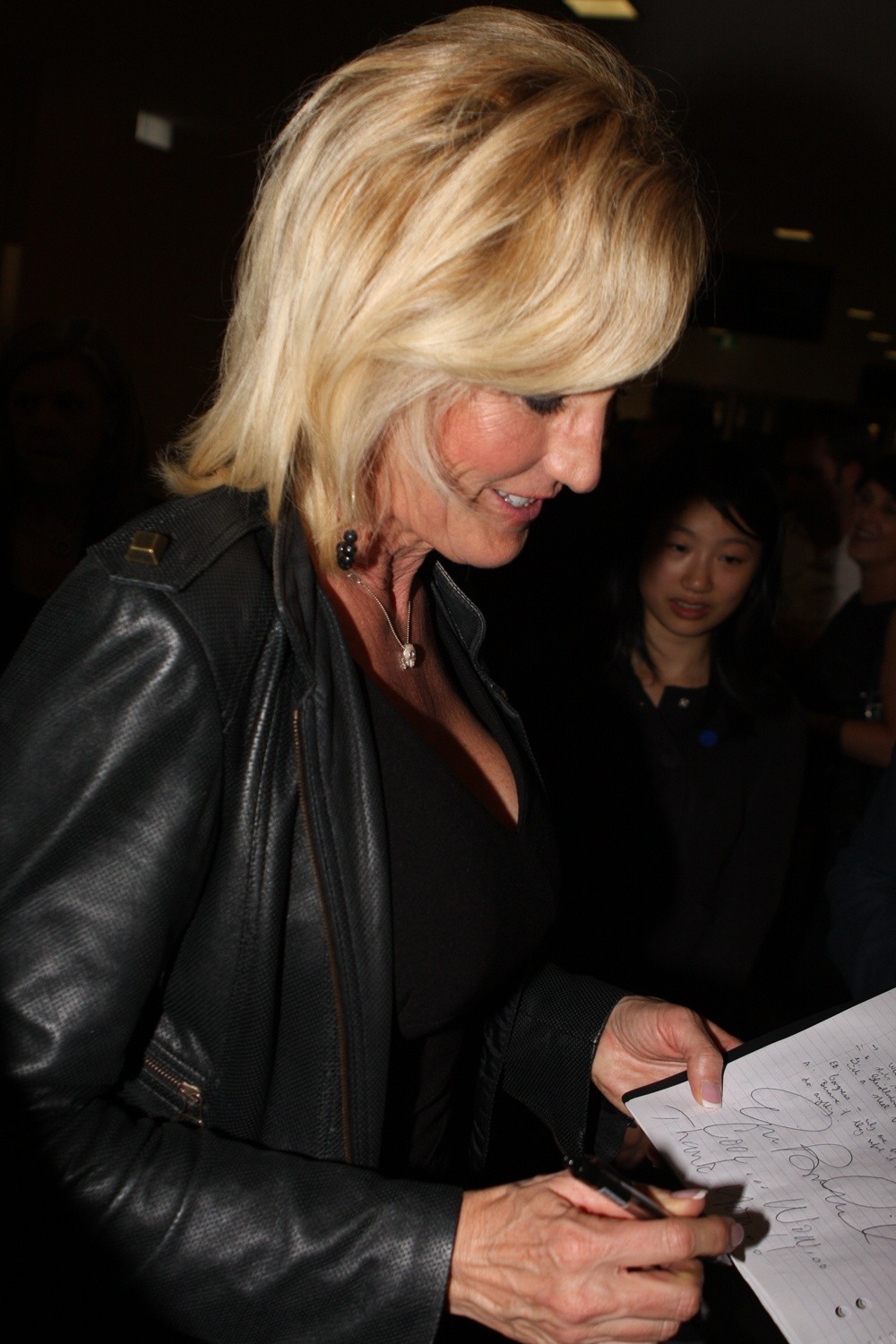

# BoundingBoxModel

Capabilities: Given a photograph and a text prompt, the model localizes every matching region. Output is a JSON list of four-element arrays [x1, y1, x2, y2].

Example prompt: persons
[[0, 319, 150, 663], [537, 412, 896, 1032], [0, 10, 745, 1344]]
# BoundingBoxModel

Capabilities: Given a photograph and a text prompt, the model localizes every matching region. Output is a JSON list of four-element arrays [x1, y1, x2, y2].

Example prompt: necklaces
[[348, 573, 416, 671]]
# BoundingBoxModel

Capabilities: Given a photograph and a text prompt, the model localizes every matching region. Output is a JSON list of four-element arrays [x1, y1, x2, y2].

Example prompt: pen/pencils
[[564, 1154, 732, 1266]]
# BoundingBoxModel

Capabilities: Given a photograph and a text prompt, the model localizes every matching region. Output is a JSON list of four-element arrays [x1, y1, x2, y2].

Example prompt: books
[[621, 983, 896, 1344]]
